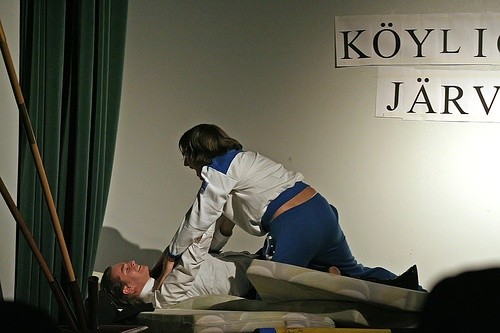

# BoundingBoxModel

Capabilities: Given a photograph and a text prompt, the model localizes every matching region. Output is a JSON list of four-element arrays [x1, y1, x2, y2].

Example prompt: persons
[[152, 123, 430, 292], [101, 204, 339, 302]]
